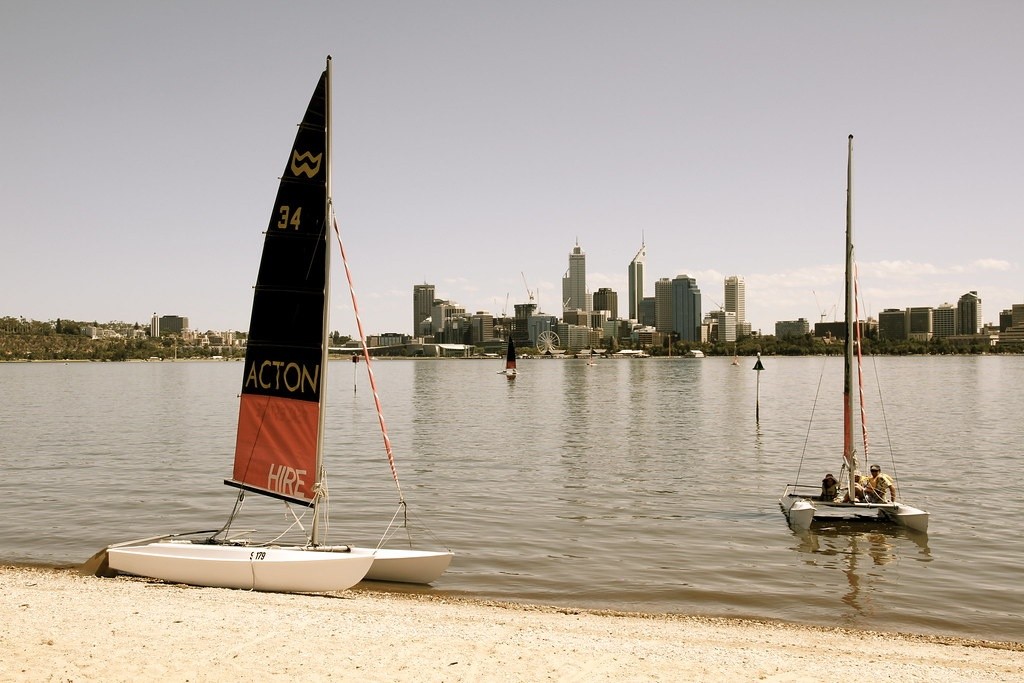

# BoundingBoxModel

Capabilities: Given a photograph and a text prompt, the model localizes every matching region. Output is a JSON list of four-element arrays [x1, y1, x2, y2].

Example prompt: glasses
[[871, 470, 878, 472]]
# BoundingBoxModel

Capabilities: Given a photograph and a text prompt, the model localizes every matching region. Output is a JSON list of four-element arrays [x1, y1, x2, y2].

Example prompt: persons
[[820, 464, 896, 504]]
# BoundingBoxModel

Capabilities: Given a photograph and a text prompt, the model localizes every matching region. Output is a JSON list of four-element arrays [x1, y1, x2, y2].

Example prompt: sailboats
[[496, 334, 518, 380], [85, 52, 458, 591], [777, 133, 934, 542]]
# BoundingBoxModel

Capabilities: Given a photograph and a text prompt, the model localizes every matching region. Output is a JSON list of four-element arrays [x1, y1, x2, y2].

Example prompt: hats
[[870, 465, 881, 471], [822, 474, 837, 485]]
